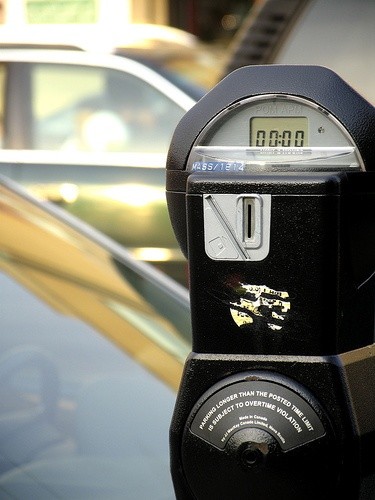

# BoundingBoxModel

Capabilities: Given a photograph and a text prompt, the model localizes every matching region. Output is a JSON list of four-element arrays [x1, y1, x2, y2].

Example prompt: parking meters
[[166, 65, 374, 500]]
